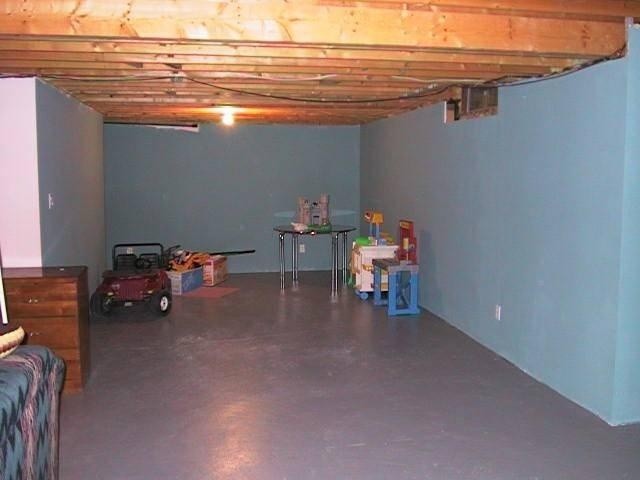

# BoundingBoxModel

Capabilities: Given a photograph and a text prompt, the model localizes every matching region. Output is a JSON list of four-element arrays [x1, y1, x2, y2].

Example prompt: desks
[[273, 226, 356, 298]]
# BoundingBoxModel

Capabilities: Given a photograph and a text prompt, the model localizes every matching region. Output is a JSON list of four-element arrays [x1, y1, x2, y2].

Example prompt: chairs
[[116, 254, 160, 271]]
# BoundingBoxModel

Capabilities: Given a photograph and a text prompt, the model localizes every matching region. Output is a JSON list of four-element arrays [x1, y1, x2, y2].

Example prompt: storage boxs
[[166, 254, 227, 295]]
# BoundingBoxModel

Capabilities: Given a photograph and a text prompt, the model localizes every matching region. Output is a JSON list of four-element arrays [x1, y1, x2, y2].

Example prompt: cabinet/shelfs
[[1, 267, 90, 395]]
